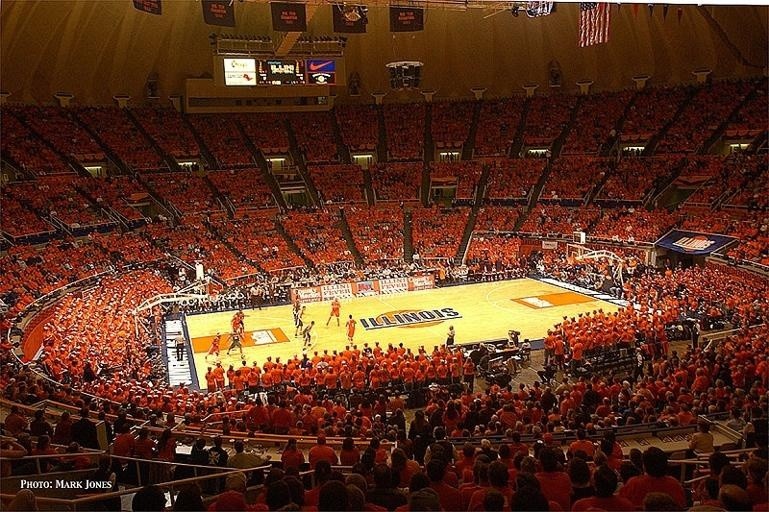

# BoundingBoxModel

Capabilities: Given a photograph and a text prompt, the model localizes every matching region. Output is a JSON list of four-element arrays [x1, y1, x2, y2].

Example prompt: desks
[[455, 338, 519, 357]]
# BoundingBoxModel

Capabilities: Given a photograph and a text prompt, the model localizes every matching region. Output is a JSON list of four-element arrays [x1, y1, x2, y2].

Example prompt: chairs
[[584, 346, 669, 375], [520, 347, 531, 362], [477, 356, 504, 381]]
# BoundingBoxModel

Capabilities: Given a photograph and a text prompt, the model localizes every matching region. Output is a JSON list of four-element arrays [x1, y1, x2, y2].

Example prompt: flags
[[577, 2, 611, 48]]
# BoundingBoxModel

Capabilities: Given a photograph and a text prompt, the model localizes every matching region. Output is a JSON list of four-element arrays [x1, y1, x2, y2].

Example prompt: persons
[[1, 74, 769, 512]]
